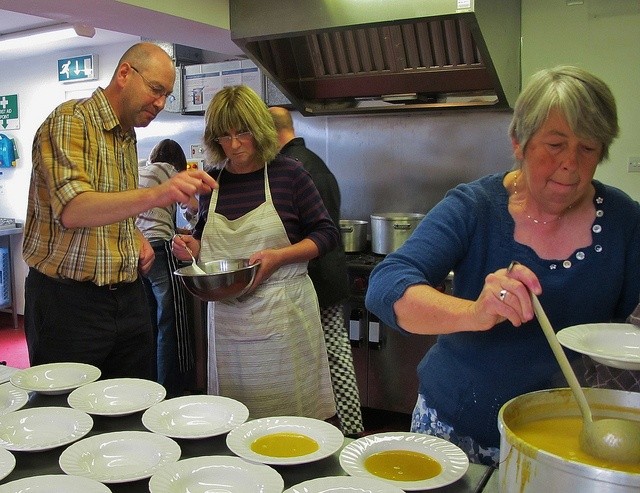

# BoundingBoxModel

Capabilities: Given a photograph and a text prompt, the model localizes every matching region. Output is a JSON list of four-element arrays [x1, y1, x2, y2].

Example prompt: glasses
[[129, 65, 176, 103], [216, 130, 252, 144]]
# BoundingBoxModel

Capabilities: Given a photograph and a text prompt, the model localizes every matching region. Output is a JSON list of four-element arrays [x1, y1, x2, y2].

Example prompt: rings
[[498, 288, 509, 301]]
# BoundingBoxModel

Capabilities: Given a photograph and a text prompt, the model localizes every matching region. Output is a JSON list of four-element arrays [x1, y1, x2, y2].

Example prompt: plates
[[142, 394, 250, 439], [0, 406, 94, 453], [149, 455, 285, 492], [0, 447, 17, 481], [59, 431, 182, 482], [283, 476, 406, 493], [11, 361, 101, 396], [225, 416, 345, 466], [339, 431, 469, 491], [0, 382, 29, 412], [1, 474, 111, 493], [556, 322, 640, 370], [68, 378, 167, 416]]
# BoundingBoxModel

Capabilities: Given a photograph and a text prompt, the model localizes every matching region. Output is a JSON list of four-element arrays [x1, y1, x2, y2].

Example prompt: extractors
[[228, 0, 523, 118]]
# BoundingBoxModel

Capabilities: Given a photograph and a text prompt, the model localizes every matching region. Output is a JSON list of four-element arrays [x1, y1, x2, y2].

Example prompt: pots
[[338, 218, 370, 254], [370, 213, 424, 256], [498, 387, 640, 493]]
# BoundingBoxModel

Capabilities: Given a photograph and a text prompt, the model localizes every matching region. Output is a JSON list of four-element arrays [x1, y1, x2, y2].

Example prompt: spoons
[[526, 287, 640, 462], [176, 234, 206, 274]]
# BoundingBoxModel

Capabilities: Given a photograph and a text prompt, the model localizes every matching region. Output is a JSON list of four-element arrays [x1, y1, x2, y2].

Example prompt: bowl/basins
[[174, 258, 262, 302]]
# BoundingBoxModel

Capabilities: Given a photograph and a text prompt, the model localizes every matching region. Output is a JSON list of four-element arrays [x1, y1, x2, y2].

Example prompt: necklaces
[[520, 202, 576, 225]]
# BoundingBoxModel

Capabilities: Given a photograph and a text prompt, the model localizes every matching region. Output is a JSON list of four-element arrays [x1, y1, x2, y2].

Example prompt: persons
[[265, 105, 364, 438], [137, 137, 200, 393], [23, 41, 177, 382], [171, 85, 343, 427], [363, 66, 640, 468]]
